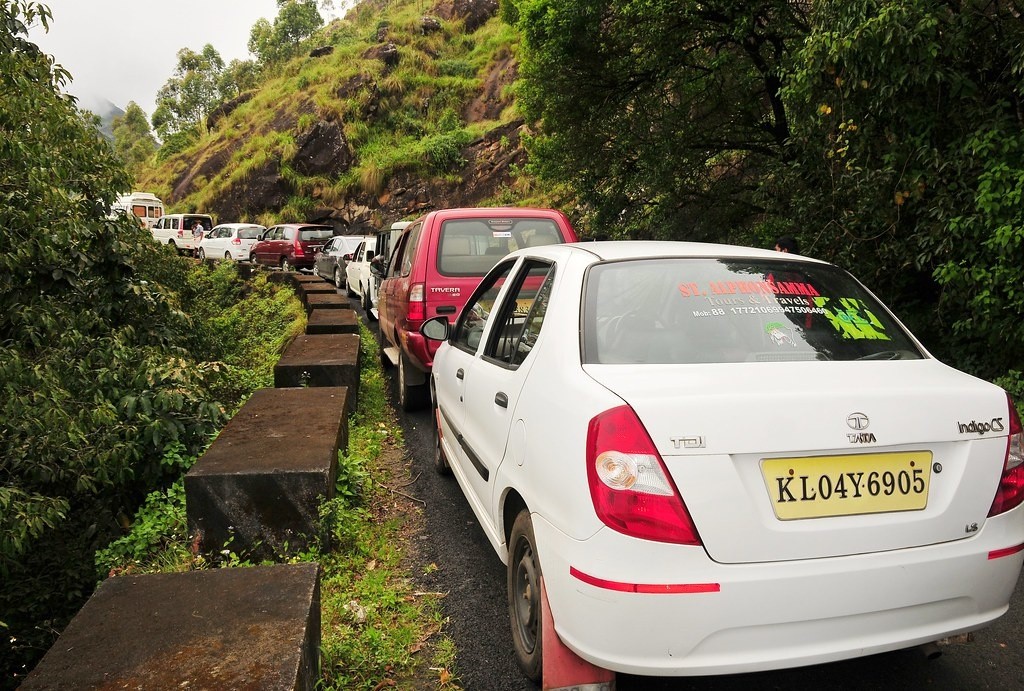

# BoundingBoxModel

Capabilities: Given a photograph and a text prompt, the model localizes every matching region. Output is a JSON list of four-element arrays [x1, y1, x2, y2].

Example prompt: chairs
[[486, 247, 510, 255]]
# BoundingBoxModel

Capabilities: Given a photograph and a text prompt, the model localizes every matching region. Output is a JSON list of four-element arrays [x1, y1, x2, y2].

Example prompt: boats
[[419, 242, 1024, 691]]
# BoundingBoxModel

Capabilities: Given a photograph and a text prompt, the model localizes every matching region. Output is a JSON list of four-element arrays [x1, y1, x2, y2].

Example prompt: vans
[[368, 208, 579, 416]]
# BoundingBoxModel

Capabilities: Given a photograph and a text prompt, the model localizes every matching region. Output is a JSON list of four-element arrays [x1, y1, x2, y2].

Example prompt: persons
[[372, 254, 380, 262], [191, 220, 203, 258]]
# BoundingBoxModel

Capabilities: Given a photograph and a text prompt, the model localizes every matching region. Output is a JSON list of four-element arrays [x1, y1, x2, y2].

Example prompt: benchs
[[442, 235, 560, 272]]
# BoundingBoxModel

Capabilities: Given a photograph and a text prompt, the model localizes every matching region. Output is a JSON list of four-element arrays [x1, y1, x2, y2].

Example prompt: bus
[[96, 191, 165, 229]]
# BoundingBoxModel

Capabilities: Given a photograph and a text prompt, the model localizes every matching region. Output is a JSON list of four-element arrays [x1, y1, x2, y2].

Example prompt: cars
[[149, 213, 215, 254], [198, 223, 267, 262], [342, 237, 379, 310], [312, 235, 376, 288], [368, 221, 415, 317], [250, 223, 335, 269]]
